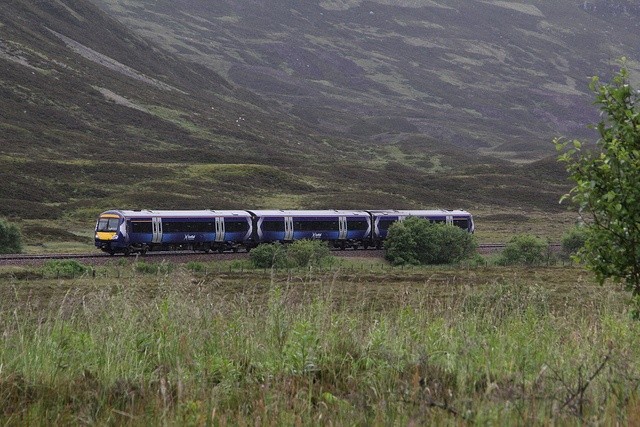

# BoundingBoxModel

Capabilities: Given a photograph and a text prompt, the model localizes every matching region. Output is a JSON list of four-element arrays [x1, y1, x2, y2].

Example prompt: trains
[[92, 207, 479, 258]]
